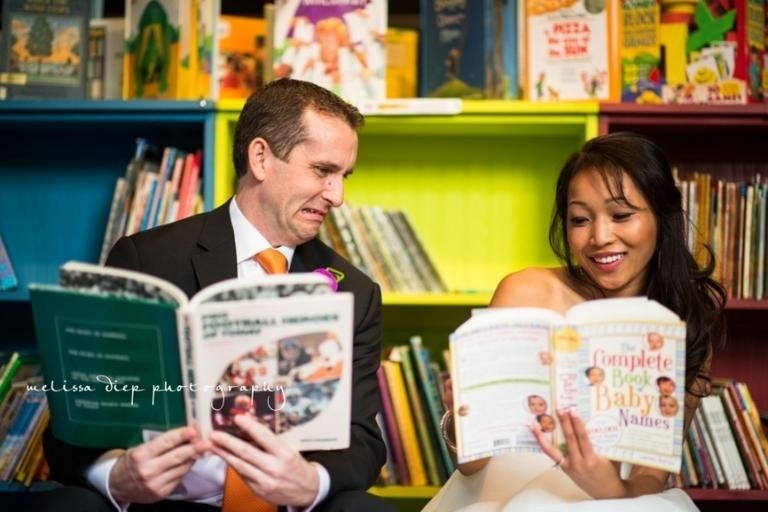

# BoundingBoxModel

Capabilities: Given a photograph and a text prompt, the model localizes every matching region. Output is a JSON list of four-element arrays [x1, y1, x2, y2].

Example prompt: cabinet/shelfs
[[0, 95, 767, 512]]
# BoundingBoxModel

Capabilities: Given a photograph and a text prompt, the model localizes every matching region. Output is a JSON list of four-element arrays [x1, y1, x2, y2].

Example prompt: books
[[326, 205, 448, 295], [23, 261, 352, 453], [1, 350, 48, 484], [671, 381, 768, 491], [667, 160, 768, 299], [100, 138, 203, 267], [448, 296, 687, 478], [0, 1, 767, 105], [378, 332, 455, 487]]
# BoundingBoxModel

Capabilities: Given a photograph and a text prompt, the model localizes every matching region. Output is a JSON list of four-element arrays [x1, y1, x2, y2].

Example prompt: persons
[[527, 333, 678, 431], [41, 79, 387, 512], [425, 130, 721, 512]]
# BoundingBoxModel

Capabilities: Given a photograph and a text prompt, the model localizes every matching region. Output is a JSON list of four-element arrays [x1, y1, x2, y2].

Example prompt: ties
[[222, 251, 289, 510]]
